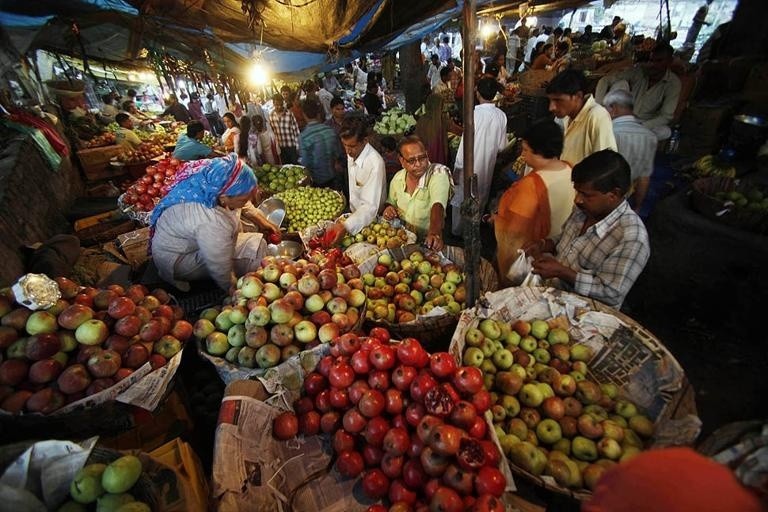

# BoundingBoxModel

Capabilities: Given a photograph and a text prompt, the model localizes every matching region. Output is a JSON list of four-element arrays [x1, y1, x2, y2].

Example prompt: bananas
[[692, 154, 737, 179]]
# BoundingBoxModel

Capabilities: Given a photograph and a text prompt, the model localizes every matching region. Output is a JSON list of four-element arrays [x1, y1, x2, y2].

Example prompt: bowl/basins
[[45, 78, 86, 98], [255, 197, 289, 234], [266, 241, 303, 265], [159, 120, 172, 127]]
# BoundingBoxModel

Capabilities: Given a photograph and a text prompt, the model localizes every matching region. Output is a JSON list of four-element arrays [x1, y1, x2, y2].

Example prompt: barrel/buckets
[[446, 285, 702, 501], [360, 239, 499, 345], [257, 198, 289, 229], [277, 240, 304, 260]]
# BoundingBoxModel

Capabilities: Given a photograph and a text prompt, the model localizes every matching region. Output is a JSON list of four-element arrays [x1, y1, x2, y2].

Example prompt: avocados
[[55, 454, 151, 512]]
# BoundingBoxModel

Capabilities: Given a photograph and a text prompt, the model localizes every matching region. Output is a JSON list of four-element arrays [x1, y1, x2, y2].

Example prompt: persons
[[147, 153, 284, 296], [158, 16, 698, 313], [123, 101, 154, 128], [108, 92, 122, 108], [142, 92, 150, 103], [114, 113, 152, 142], [101, 95, 120, 117], [120, 89, 137, 107]]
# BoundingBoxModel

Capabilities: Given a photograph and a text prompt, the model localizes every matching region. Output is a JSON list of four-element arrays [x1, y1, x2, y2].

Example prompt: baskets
[[365, 313, 456, 350]]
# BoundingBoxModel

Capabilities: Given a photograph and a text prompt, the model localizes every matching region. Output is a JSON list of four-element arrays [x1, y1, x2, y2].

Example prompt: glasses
[[400, 153, 427, 164]]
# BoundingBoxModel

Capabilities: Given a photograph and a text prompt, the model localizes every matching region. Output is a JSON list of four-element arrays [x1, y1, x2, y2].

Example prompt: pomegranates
[[121, 158, 189, 210]]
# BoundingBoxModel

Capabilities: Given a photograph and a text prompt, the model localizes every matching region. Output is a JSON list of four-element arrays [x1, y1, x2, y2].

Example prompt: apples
[[271, 186, 467, 323], [463, 318, 651, 489], [194, 248, 367, 368], [274, 327, 506, 511], [1, 276, 193, 412]]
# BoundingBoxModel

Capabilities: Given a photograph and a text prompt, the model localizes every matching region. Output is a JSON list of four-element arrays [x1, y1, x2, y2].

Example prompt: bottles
[[664, 123, 681, 155]]
